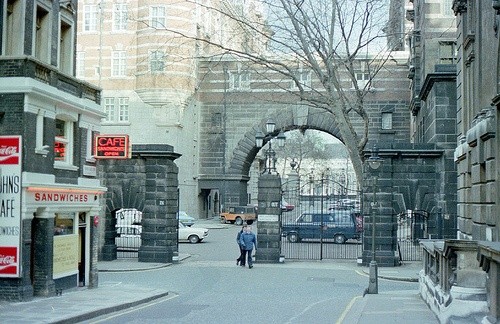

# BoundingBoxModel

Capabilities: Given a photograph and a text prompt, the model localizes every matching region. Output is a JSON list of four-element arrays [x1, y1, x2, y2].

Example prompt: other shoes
[[236, 259, 239, 265], [242, 265, 245, 267], [249, 265, 253, 269]]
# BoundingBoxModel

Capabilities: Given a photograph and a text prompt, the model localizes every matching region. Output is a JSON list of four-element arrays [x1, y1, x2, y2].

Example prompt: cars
[[329, 199, 361, 211], [281, 200, 294, 212], [179, 211, 209, 244], [115, 224, 142, 252]]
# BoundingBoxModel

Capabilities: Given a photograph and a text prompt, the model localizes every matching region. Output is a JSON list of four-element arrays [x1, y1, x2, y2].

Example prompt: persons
[[236, 227, 246, 266], [240, 226, 257, 268]]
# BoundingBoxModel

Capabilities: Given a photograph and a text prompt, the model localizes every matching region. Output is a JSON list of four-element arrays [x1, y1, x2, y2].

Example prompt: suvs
[[220, 206, 256, 226], [282, 210, 361, 244]]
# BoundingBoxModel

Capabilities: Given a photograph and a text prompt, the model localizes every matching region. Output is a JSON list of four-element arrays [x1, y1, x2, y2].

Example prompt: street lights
[[365, 145, 384, 294]]
